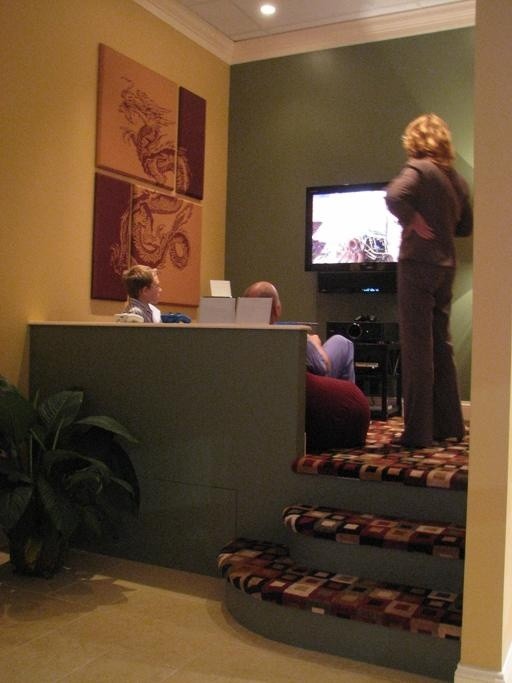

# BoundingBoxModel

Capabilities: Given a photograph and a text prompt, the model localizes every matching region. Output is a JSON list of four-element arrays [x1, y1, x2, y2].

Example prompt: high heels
[[390, 424, 466, 449]]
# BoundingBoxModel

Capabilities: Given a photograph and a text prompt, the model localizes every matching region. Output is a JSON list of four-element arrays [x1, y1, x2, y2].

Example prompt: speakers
[[324, 320, 400, 343]]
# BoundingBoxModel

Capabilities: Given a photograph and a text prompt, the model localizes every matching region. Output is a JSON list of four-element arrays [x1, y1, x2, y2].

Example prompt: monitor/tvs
[[304, 182, 404, 275]]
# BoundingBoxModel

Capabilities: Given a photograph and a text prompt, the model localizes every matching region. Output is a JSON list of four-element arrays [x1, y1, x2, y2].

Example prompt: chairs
[[306, 371, 371, 450]]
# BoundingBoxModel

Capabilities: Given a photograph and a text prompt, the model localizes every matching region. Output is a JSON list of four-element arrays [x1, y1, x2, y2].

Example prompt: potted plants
[[0, 377, 145, 578]]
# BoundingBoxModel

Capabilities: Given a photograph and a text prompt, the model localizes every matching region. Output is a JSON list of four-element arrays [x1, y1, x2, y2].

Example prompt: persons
[[122, 263, 165, 323], [381, 111, 475, 450], [240, 279, 356, 386]]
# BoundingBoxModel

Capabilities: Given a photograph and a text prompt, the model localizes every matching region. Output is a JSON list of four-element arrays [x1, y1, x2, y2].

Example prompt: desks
[[355, 342, 402, 421]]
